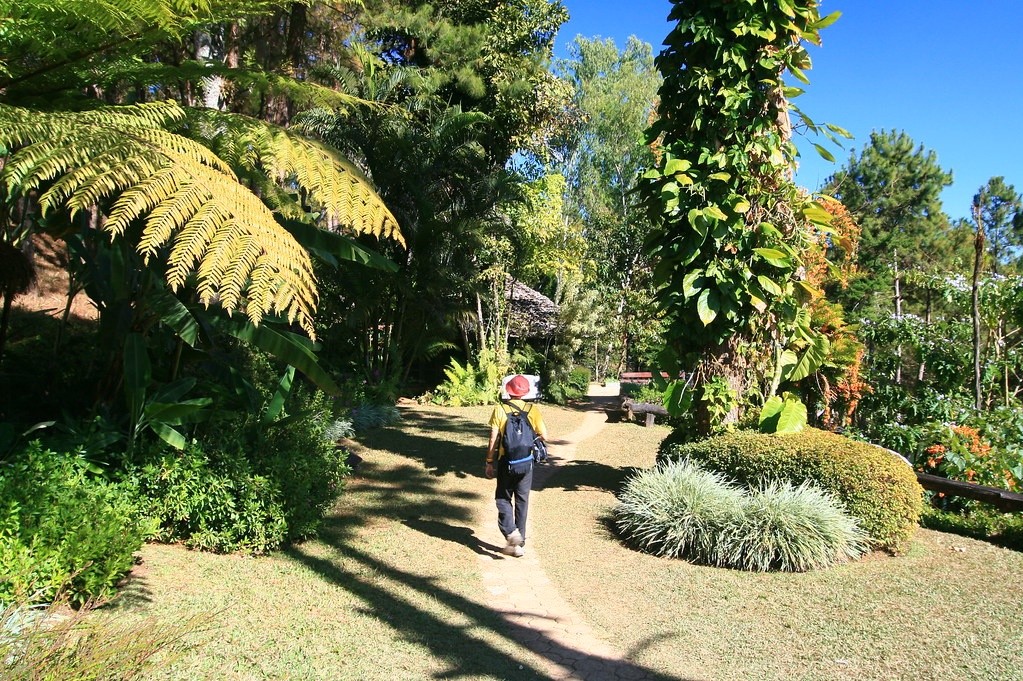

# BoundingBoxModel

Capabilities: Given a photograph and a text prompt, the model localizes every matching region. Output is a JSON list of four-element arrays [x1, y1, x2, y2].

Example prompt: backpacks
[[501, 402, 533, 477]]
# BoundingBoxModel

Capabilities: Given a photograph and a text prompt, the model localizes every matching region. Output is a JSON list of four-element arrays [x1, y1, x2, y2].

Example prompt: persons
[[485, 375, 547, 556]]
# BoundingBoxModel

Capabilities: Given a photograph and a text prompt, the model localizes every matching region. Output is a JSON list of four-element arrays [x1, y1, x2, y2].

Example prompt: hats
[[507, 374, 530, 396]]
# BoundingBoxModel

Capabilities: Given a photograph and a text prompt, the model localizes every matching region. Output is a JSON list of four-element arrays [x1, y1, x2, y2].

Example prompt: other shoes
[[514, 544, 525, 557], [502, 527, 522, 555]]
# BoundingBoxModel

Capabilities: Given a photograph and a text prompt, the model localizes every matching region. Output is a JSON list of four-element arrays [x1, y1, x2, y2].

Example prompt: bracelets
[[486, 461, 493, 464]]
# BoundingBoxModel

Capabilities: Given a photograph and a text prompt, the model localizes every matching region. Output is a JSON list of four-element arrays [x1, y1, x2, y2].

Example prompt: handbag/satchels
[[533, 433, 547, 464]]
